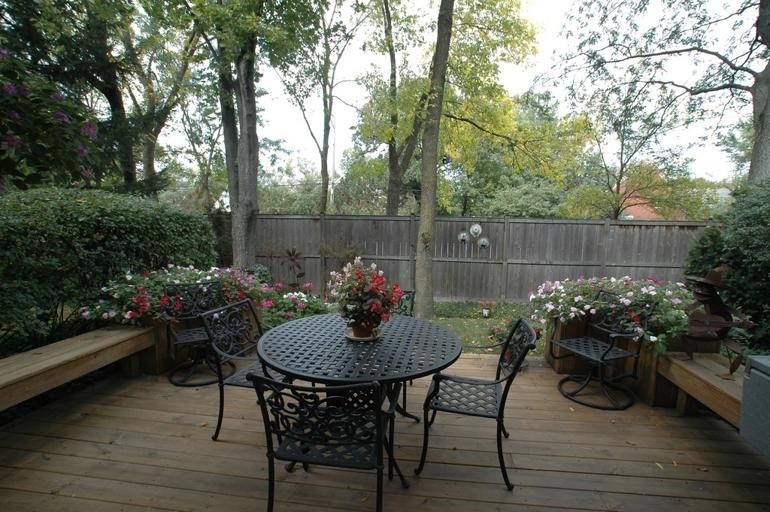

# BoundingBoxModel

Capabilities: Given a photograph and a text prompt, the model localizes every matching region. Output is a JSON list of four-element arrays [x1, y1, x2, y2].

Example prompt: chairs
[[244, 372, 409, 512], [549, 290, 658, 410], [161, 281, 239, 387], [389, 290, 416, 413], [413, 318, 536, 491], [198, 298, 296, 448]]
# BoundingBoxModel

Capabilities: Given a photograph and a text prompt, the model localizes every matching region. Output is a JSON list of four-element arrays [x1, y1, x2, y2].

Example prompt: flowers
[[326, 255, 405, 322]]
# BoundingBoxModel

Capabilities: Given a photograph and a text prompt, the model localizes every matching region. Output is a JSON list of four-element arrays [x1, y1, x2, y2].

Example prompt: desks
[[255, 313, 463, 489]]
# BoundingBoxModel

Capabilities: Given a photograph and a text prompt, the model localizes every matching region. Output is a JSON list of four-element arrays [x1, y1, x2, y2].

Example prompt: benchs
[[0, 324, 166, 415], [656, 352, 747, 431]]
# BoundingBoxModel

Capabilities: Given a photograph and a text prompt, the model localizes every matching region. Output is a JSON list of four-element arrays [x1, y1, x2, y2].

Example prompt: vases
[[343, 320, 384, 342]]
